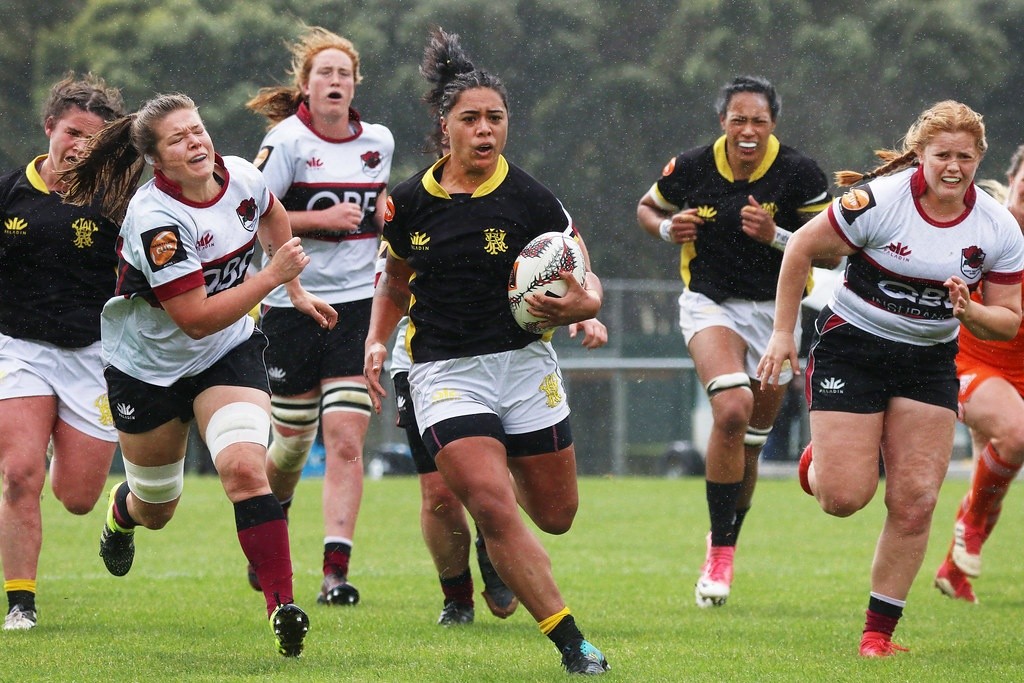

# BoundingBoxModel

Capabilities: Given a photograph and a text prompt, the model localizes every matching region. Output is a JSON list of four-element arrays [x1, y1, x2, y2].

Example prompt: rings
[[546, 314, 550, 319]]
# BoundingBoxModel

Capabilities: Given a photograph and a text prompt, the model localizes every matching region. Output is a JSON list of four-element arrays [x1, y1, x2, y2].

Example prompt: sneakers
[[438, 602, 475, 626], [953, 521, 989, 577], [1, 603, 38, 632], [318, 573, 358, 607], [45, 437, 54, 461], [473, 523, 520, 619], [934, 561, 980, 604], [270, 592, 309, 656], [798, 443, 813, 496], [857, 633, 909, 659], [560, 639, 611, 676], [100, 481, 136, 576], [695, 533, 737, 607], [247, 563, 263, 592]]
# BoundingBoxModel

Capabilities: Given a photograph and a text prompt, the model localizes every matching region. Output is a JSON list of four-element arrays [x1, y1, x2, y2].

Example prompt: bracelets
[[660, 220, 672, 242], [771, 226, 793, 252]]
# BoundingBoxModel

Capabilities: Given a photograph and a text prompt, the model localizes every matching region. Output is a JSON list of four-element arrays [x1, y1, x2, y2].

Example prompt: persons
[[935, 148, 1024, 602], [391, 314, 607, 624], [361, 29, 609, 676], [637, 76, 840, 605], [755, 101, 1024, 657], [0, 73, 126, 629], [54, 94, 337, 656], [249, 30, 395, 606]]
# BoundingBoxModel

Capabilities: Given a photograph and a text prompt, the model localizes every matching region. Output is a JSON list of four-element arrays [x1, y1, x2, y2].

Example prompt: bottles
[[368, 453, 384, 480]]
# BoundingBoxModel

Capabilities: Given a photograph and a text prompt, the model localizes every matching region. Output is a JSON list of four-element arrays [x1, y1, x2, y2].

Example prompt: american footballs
[[508, 227, 584, 334]]
